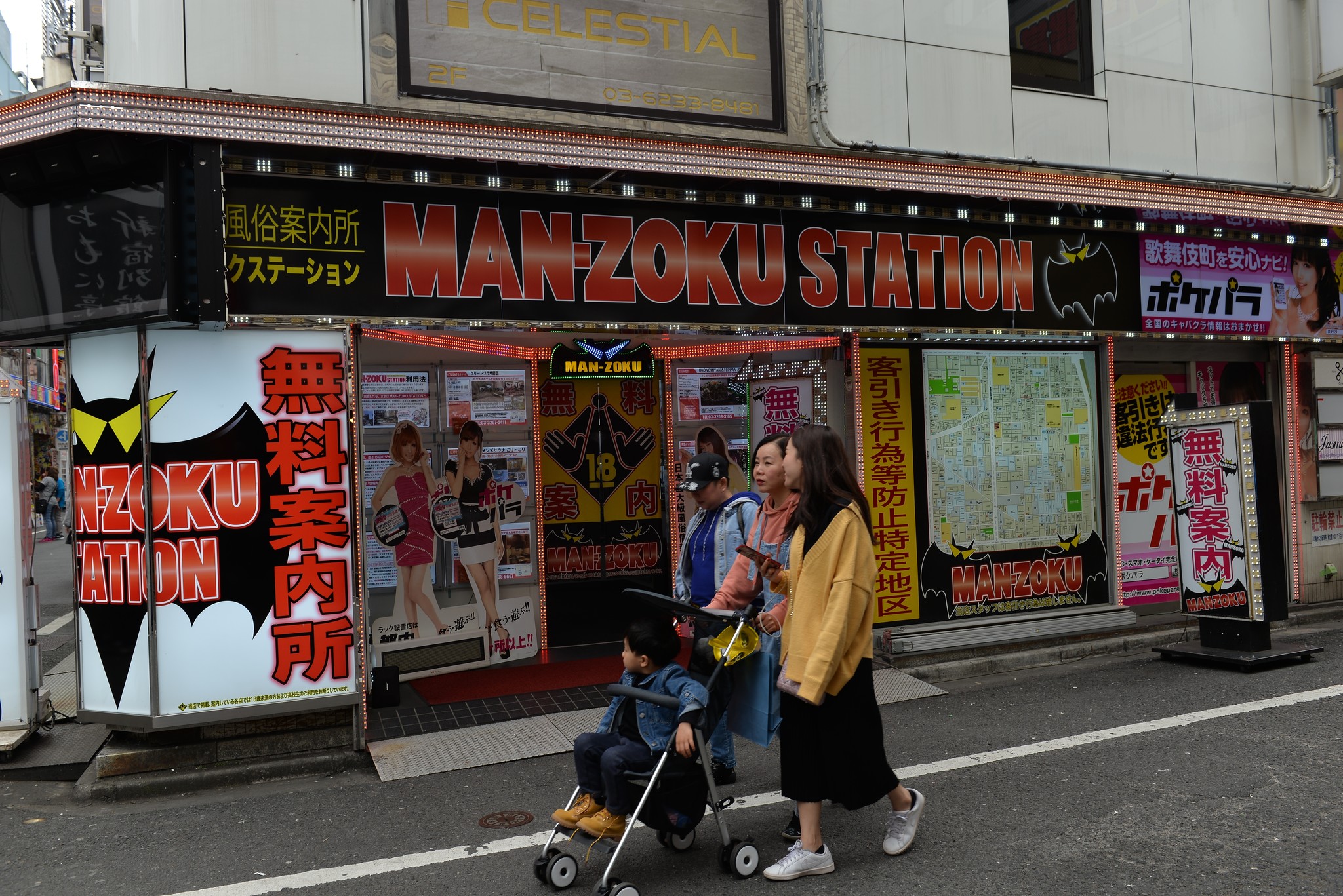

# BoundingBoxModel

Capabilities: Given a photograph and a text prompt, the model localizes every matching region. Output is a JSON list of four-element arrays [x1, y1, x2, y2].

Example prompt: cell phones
[[735, 544, 781, 570]]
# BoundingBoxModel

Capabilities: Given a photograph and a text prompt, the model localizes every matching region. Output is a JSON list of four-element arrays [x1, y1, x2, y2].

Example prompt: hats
[[674, 452, 729, 492]]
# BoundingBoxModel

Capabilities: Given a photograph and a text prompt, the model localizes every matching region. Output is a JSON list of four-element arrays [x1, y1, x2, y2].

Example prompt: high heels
[[498, 628, 510, 660], [488, 634, 492, 657]]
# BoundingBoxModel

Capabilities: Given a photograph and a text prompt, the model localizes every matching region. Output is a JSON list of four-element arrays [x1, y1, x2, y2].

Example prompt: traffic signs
[[55, 427, 68, 451]]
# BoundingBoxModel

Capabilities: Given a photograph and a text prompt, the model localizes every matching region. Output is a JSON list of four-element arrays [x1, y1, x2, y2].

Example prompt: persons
[[552, 618, 708, 840], [51, 467, 65, 540], [371, 417, 451, 640], [1264, 246, 1343, 336], [675, 452, 765, 785], [1296, 364, 1318, 501], [30, 465, 58, 543], [699, 433, 802, 840], [63, 481, 72, 533], [756, 422, 925, 879], [444, 419, 512, 662]]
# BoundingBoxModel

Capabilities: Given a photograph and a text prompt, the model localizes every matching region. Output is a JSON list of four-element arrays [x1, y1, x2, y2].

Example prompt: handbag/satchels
[[65, 529, 72, 545], [777, 651, 826, 707], [35, 498, 48, 514]]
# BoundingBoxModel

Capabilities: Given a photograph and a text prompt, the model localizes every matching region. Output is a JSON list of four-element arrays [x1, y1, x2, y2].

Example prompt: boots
[[551, 792, 605, 830], [576, 807, 626, 842]]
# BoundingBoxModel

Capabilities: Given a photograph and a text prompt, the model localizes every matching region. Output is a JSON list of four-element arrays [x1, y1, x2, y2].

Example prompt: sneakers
[[39, 536, 56, 543], [781, 810, 802, 839], [882, 787, 925, 856], [55, 532, 64, 540], [709, 758, 736, 785], [764, 839, 835, 880]]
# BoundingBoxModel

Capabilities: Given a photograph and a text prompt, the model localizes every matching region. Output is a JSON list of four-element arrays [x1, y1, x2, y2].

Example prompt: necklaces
[[401, 463, 416, 473], [1297, 298, 1318, 323], [1299, 449, 1308, 477]]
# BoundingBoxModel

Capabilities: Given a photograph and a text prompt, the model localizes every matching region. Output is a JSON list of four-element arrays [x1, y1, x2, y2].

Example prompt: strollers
[[533, 587, 765, 896]]
